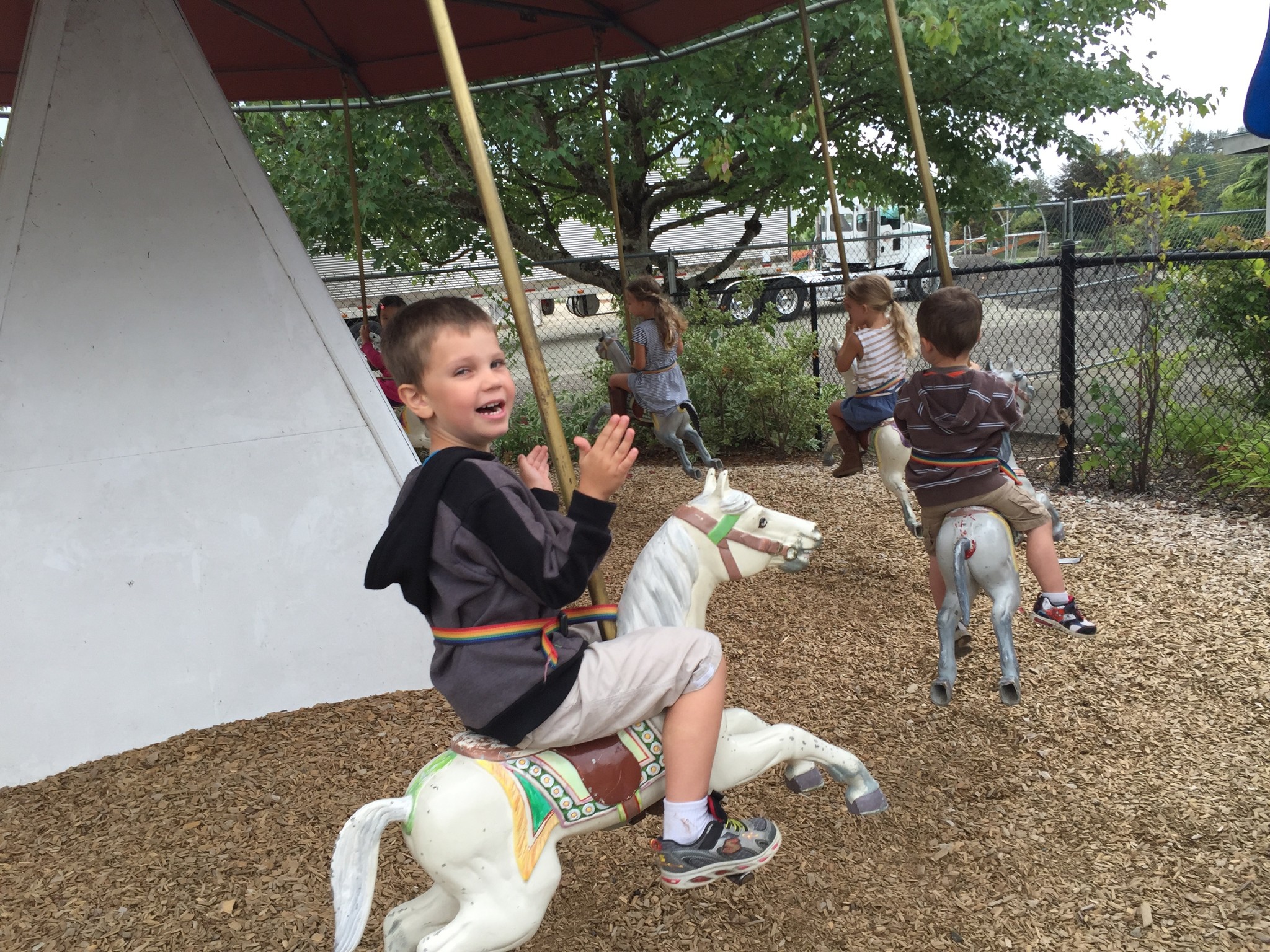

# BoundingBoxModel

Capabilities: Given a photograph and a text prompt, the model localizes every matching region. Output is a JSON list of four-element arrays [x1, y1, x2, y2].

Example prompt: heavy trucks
[[305, 166, 951, 372]]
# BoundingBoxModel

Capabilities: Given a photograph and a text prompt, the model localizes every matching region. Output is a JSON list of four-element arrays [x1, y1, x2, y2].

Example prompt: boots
[[832, 424, 864, 478], [608, 386, 636, 443]]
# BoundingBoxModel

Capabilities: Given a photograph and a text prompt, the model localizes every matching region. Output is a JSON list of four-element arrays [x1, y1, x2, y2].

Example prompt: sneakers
[[1029, 592, 1097, 638], [955, 618, 973, 659], [649, 788, 782, 890]]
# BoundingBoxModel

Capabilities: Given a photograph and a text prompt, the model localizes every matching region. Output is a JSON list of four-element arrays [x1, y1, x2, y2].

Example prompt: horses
[[586, 329, 724, 479], [930, 367, 1067, 705], [822, 335, 924, 540], [328, 467, 889, 952]]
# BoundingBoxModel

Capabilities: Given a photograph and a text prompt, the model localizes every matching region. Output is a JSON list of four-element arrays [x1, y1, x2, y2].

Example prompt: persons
[[895, 285, 1098, 660], [364, 295, 784, 890], [359, 295, 410, 408], [829, 274, 915, 480], [608, 274, 690, 423]]
